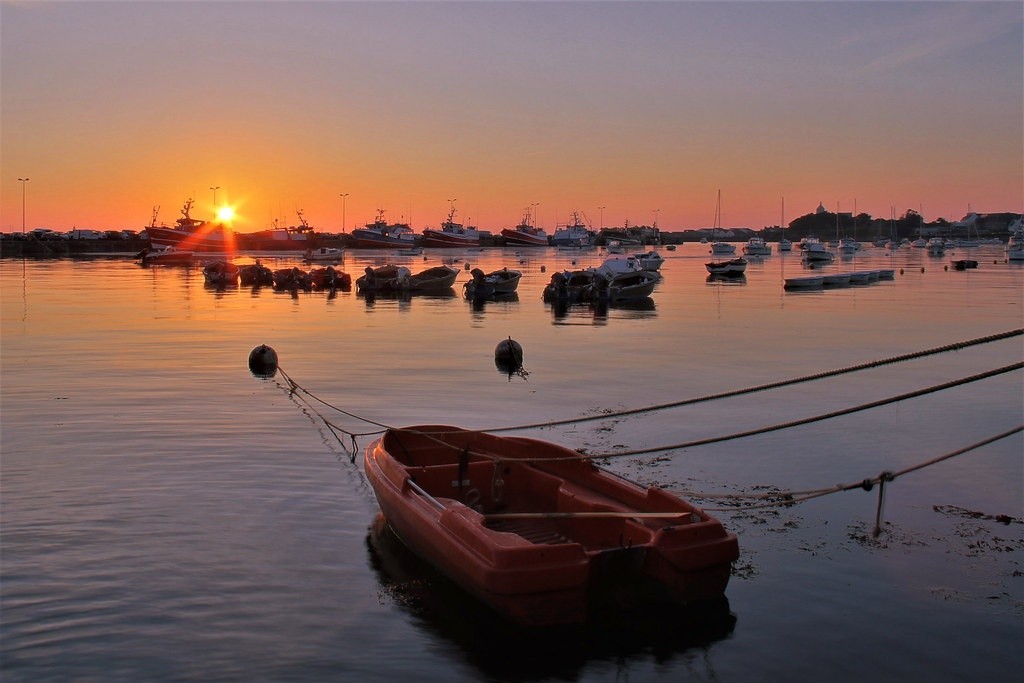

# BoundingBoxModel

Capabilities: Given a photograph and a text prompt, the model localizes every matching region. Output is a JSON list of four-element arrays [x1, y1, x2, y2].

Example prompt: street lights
[[339, 193, 349, 234], [597, 206, 605, 231], [209, 185, 220, 219], [17, 176, 31, 235], [531, 202, 540, 229], [652, 209, 660, 227], [447, 197, 456, 221]]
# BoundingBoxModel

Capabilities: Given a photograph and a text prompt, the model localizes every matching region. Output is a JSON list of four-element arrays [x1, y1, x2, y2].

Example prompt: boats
[[356, 263, 461, 289], [144, 219, 647, 253], [632, 251, 665, 271], [543, 253, 658, 303], [272, 269, 309, 289], [666, 211, 1024, 293], [238, 266, 272, 284], [367, 424, 739, 624], [309, 268, 352, 290], [203, 259, 238, 284], [461, 270, 523, 300]]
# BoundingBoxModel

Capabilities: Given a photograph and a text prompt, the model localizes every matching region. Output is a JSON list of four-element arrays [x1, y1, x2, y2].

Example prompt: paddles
[[482, 510, 693, 518]]
